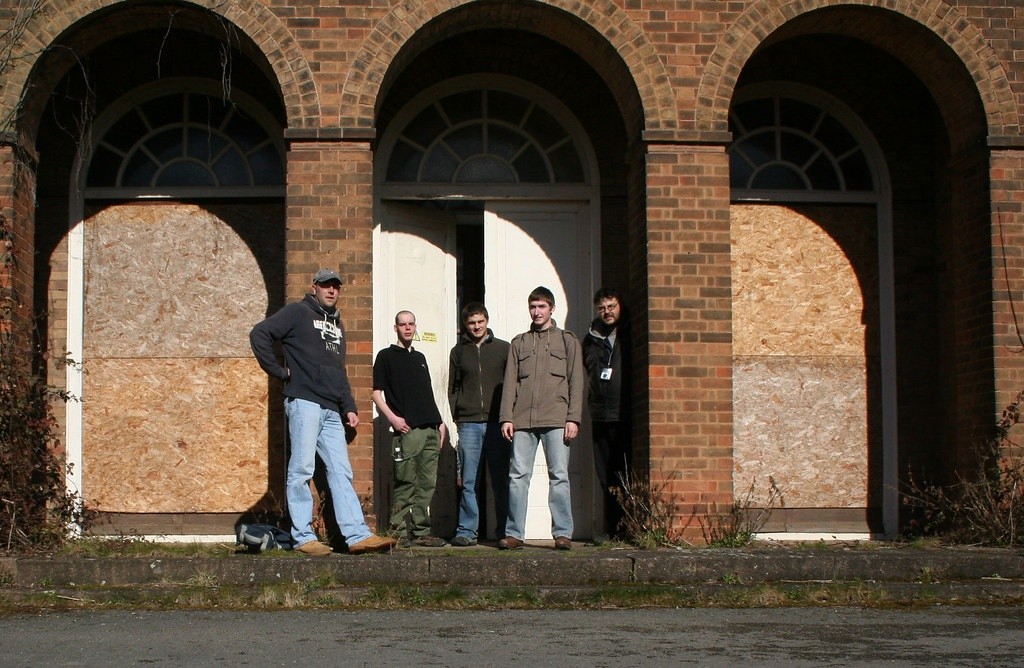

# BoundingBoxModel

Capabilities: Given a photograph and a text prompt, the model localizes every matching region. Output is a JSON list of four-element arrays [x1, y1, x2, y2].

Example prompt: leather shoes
[[499, 537, 523, 549], [556, 537, 572, 550]]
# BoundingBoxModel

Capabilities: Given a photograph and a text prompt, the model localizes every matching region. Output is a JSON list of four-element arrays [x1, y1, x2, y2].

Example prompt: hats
[[312, 268, 342, 287]]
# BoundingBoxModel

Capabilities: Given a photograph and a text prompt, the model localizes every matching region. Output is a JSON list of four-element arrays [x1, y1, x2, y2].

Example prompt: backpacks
[[236, 523, 292, 552]]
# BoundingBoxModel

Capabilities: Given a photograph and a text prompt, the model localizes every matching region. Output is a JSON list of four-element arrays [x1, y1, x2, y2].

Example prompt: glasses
[[597, 301, 619, 311]]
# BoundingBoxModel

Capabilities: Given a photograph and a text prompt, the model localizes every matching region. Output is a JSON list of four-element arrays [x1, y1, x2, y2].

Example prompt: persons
[[249, 269, 398, 558], [447, 300, 511, 547], [372, 309, 450, 547], [498, 284, 585, 549], [582, 287, 630, 545]]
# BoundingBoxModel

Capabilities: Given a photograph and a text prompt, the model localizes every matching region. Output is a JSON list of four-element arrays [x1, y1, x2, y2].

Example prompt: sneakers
[[451, 537, 478, 546], [294, 541, 332, 556], [414, 536, 446, 547], [349, 536, 397, 554], [396, 538, 412, 548]]
